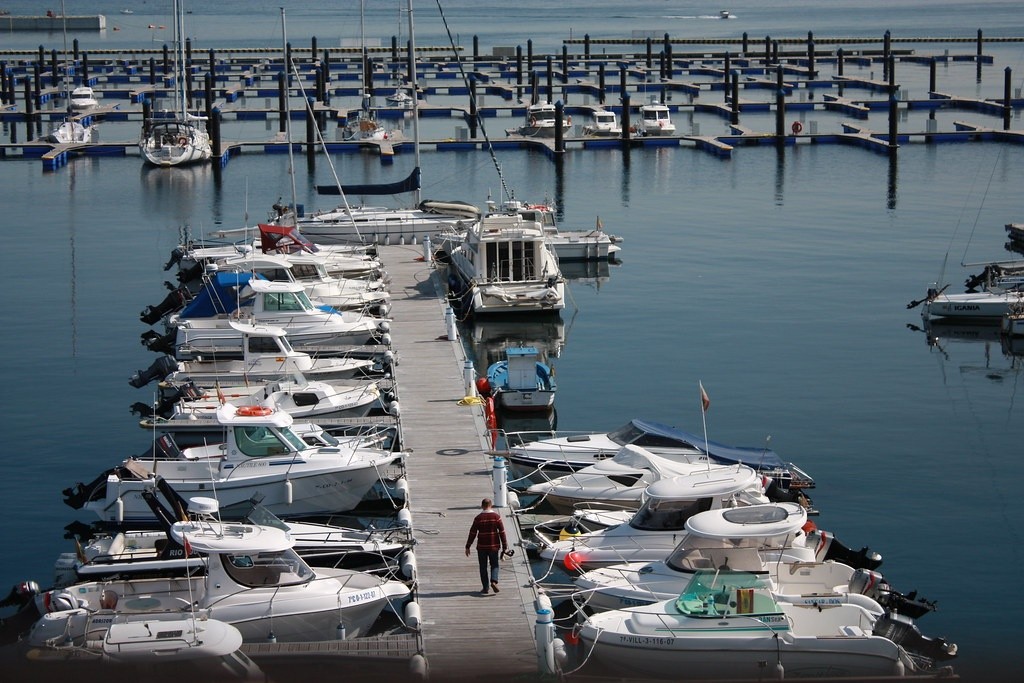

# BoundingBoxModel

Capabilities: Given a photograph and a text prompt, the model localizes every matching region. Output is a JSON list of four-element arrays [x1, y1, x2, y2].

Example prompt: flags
[[183, 535, 192, 559], [699, 383, 709, 410]]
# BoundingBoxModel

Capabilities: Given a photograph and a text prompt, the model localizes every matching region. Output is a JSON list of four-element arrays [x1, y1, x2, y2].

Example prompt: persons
[[465, 498, 510, 594]]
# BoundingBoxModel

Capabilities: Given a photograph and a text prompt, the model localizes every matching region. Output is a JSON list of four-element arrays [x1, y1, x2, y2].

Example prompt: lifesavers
[[530, 205, 546, 211], [531, 117, 536, 126], [792, 122, 802, 132], [179, 137, 187, 144], [45, 590, 55, 612], [237, 406, 271, 416], [485, 397, 495, 448]]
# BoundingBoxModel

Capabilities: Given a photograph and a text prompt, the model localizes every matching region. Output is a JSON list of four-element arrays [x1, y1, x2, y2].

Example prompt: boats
[[467, 316, 568, 371], [533, 380, 883, 570], [499, 407, 560, 437], [435, 166, 626, 260], [450, 166, 568, 316], [924, 222, 1024, 338], [126, 223, 397, 403], [67, 86, 98, 110], [528, 442, 818, 516], [486, 346, 558, 415], [634, 98, 676, 137], [22, 547, 413, 643], [207, 58, 485, 244], [518, 99, 573, 139], [60, 392, 409, 531], [574, 501, 936, 623], [581, 106, 620, 137], [16, 591, 268, 683], [579, 566, 958, 683], [484, 417, 790, 486], [719, 10, 730, 18], [561, 260, 611, 282], [55, 497, 414, 582], [140, 337, 381, 433]]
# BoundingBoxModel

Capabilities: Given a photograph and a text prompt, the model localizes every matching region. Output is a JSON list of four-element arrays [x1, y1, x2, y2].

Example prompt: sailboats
[[340, 0, 388, 146], [385, 1, 414, 107], [137, 0, 212, 168], [37, 0, 98, 143]]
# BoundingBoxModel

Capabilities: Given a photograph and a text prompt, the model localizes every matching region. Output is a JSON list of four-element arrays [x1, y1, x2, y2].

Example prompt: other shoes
[[481, 589, 488, 594], [491, 582, 500, 593]]
